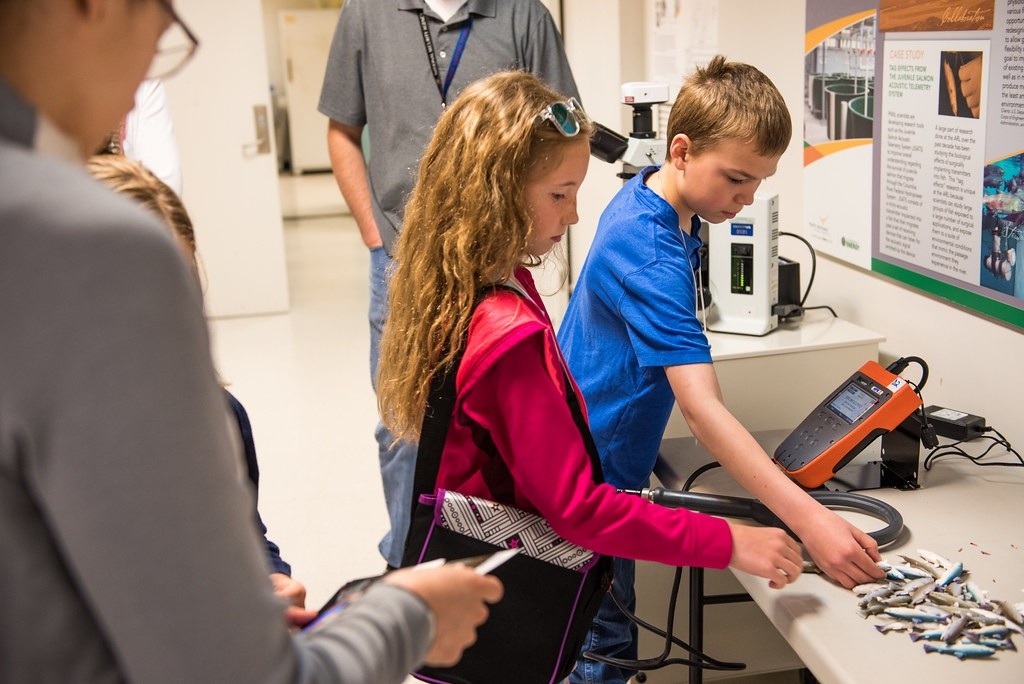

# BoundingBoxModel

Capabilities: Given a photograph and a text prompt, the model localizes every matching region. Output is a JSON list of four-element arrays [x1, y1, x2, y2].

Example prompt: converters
[[918, 405, 985, 442]]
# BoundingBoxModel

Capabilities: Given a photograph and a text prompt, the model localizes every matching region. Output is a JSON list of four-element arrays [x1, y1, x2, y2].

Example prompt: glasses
[[533, 96, 592, 137], [142, 0, 197, 80]]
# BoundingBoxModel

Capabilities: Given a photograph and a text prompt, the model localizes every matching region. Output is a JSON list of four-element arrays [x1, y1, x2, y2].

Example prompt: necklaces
[[678, 226, 707, 335]]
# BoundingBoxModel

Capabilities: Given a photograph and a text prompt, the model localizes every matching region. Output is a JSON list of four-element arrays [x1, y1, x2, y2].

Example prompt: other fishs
[[847, 545, 1024, 662]]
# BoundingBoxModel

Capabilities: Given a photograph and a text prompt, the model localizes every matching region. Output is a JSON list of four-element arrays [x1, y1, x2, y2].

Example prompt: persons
[[320, 0, 586, 565], [557, 57, 885, 684], [375, 71, 805, 684], [0, 0, 504, 684]]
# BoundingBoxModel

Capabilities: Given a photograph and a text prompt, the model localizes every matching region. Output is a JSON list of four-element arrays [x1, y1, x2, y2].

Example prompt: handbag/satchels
[[400, 281, 615, 684]]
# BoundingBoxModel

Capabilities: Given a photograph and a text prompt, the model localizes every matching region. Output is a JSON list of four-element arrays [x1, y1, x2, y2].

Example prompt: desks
[[636, 310, 886, 684], [653, 428, 1024, 684]]
